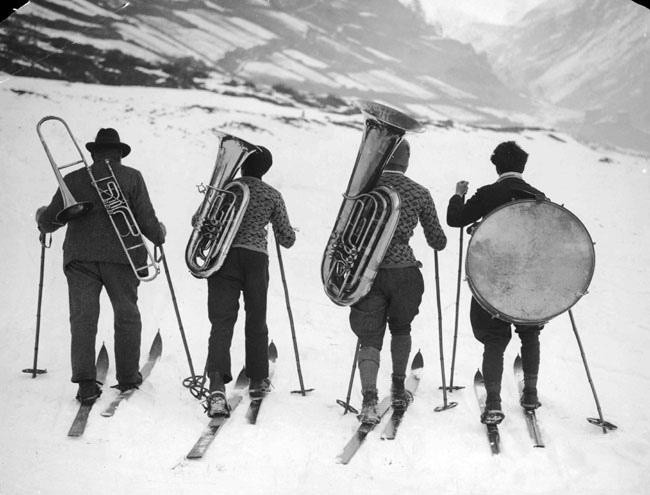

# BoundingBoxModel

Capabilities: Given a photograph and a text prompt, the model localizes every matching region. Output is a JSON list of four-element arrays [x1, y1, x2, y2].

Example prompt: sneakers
[[361, 407, 381, 425], [481, 398, 505, 425], [249, 379, 272, 401], [207, 383, 230, 418], [76, 381, 103, 406], [392, 390, 414, 413], [520, 387, 538, 409], [120, 371, 142, 395]]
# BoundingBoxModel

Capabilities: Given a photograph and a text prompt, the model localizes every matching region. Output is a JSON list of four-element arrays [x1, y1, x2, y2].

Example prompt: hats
[[86, 129, 130, 158], [243, 145, 272, 175], [491, 140, 529, 172], [387, 139, 410, 168]]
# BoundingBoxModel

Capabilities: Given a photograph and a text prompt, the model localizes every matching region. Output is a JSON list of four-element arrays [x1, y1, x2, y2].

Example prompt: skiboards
[[332, 349, 423, 467], [186, 340, 277, 459], [68, 329, 162, 437], [473, 352, 545, 455]]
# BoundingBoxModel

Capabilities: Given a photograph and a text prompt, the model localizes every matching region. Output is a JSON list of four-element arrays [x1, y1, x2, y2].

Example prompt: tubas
[[184, 127, 262, 279], [320, 100, 427, 308]]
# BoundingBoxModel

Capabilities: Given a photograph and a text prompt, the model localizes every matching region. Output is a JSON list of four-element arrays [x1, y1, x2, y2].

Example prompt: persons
[[350, 137, 448, 424], [205, 147, 296, 417], [447, 142, 552, 424], [37, 128, 166, 405]]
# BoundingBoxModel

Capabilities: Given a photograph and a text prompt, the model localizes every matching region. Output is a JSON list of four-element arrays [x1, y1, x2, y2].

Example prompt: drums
[[463, 197, 596, 327]]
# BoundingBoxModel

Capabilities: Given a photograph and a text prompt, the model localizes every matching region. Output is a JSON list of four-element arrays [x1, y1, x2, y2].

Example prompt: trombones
[[37, 115, 159, 282]]
